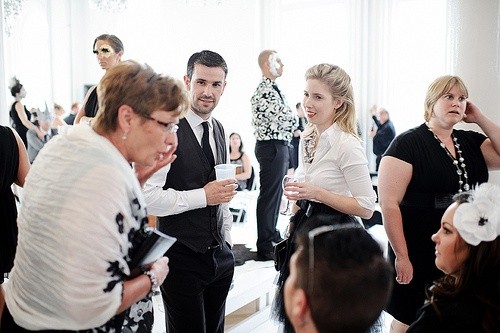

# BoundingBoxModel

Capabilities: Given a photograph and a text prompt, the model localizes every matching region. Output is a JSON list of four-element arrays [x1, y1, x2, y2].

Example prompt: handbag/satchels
[[273, 238, 288, 271]]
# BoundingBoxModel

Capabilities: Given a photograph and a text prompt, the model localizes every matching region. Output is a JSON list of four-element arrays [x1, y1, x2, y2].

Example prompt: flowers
[[453, 183, 500, 246]]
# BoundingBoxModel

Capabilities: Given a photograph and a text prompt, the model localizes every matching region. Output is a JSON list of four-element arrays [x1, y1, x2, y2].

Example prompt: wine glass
[[280, 175, 300, 216]]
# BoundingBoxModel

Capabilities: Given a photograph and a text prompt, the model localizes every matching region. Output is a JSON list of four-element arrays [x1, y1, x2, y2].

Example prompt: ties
[[201, 121, 215, 171]]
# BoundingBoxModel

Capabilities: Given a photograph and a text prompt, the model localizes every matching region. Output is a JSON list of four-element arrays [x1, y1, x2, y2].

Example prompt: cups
[[214, 164, 236, 182]]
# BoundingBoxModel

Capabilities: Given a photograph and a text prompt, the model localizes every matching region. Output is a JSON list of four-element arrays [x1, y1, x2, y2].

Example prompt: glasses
[[141, 112, 179, 135]]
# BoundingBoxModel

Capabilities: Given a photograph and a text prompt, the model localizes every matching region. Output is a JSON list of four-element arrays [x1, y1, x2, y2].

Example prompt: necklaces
[[426, 121, 470, 194]]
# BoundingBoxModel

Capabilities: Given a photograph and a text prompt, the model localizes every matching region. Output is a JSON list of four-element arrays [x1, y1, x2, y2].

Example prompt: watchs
[[142, 271, 158, 298]]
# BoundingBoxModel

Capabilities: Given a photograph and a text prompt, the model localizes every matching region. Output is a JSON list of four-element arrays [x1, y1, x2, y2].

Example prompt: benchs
[[222, 262, 282, 333]]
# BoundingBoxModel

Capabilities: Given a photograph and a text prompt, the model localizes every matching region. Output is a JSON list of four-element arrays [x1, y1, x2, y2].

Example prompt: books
[[135, 229, 176, 277]]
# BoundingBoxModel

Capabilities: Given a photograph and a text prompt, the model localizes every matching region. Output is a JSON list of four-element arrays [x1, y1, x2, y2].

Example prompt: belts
[[257, 139, 288, 146]]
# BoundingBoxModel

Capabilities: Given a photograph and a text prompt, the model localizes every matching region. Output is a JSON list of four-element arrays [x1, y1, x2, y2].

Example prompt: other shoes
[[257, 251, 275, 260], [275, 238, 283, 243]]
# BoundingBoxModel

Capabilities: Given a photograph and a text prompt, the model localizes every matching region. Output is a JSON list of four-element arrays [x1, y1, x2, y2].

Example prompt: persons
[[406, 182, 500, 333], [0, 34, 125, 283], [283, 214, 394, 333], [141, 50, 239, 333], [269, 62, 376, 333], [280, 101, 309, 216], [227, 132, 251, 191], [377, 74, 500, 333], [370, 100, 396, 202], [0, 59, 191, 333]]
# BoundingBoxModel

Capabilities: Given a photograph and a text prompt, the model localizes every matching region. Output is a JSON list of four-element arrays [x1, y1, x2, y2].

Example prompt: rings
[[298, 192, 301, 198]]
